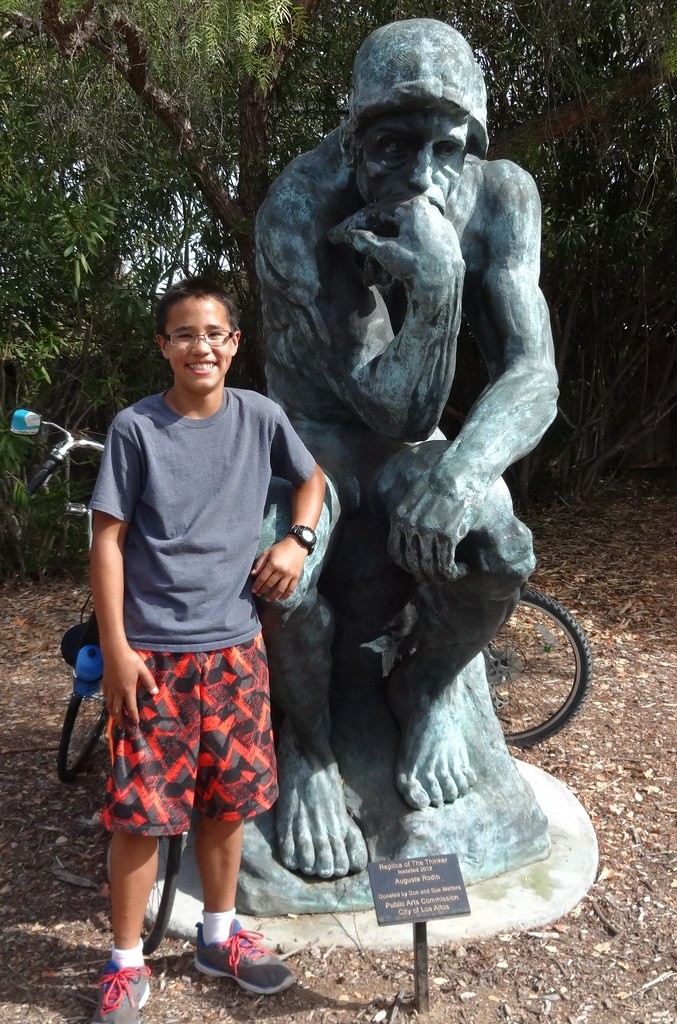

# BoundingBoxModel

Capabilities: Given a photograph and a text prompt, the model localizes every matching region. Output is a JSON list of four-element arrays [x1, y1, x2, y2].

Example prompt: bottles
[[74, 644, 103, 697]]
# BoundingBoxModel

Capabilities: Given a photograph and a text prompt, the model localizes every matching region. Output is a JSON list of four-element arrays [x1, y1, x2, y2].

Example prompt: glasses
[[162, 330, 234, 346]]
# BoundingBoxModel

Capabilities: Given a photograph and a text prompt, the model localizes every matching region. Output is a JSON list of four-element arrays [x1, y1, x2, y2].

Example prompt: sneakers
[[195, 918, 294, 994], [91, 957, 151, 1024]]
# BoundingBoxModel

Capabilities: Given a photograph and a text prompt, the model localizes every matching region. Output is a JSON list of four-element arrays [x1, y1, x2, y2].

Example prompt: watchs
[[285, 525, 317, 556]]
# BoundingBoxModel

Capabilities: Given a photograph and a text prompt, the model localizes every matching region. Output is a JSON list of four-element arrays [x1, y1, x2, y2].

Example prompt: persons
[[257, 18, 560, 878], [89, 276, 326, 1024]]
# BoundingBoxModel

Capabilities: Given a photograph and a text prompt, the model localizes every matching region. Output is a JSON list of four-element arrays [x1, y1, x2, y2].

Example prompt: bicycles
[[11, 407, 182, 956], [480, 584, 592, 750]]
